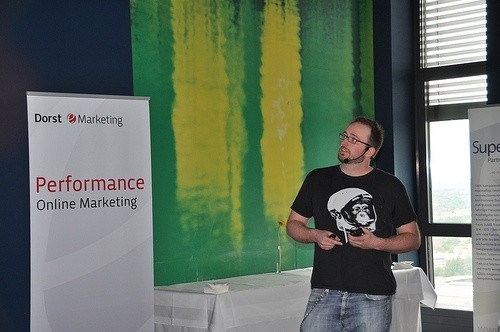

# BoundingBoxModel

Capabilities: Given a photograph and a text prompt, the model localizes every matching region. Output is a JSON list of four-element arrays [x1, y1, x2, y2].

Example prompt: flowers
[[277, 219, 285, 227]]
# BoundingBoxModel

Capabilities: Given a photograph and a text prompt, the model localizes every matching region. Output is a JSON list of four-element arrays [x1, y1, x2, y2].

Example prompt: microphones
[[344, 147, 369, 163]]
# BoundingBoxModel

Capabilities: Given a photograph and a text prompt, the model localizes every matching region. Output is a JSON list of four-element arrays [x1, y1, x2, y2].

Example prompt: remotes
[[350, 228, 364, 236]]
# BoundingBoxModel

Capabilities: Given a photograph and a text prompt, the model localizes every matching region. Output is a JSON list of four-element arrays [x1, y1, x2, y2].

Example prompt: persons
[[285, 117, 421, 332]]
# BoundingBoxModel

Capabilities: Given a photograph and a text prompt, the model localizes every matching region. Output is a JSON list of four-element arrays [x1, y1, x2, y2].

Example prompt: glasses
[[339, 133, 376, 149]]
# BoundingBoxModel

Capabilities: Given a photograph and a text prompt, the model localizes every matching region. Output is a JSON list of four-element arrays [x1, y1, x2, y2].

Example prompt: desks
[[154, 266, 438, 332]]
